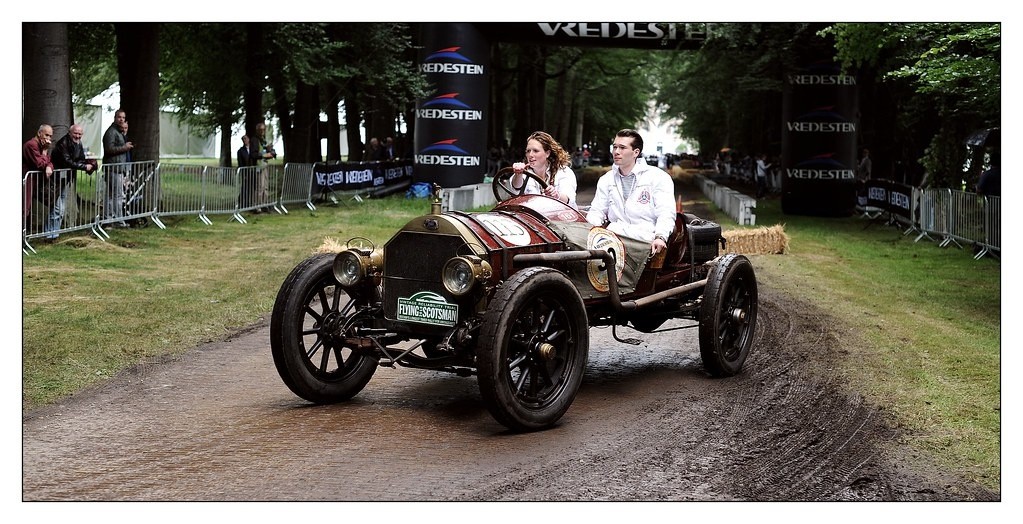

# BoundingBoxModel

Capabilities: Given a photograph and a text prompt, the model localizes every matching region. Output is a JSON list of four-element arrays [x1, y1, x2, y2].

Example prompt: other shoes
[[115, 221, 130, 227], [47, 237, 63, 244], [262, 207, 271, 212]]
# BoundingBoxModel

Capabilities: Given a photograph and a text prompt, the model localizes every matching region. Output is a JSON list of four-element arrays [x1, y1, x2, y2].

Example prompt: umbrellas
[[963, 126, 1001, 147]]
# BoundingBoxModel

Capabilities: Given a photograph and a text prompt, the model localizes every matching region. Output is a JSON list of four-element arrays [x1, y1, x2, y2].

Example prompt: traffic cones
[[675, 194, 686, 214]]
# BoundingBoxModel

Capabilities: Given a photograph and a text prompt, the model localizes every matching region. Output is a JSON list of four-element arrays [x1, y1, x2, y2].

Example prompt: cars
[[269, 167, 759, 434], [644, 154, 659, 167]]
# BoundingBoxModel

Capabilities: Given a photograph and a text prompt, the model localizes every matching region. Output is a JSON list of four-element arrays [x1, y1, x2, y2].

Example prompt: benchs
[[577, 205, 695, 295]]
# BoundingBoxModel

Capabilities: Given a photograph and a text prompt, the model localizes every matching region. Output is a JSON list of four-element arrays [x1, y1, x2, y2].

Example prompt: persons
[[22, 124, 54, 229], [509, 131, 577, 211], [238, 122, 276, 213], [44, 125, 96, 244], [976, 145, 1001, 197], [756, 154, 771, 198], [352, 137, 396, 162], [585, 128, 676, 285], [103, 110, 134, 228]]
